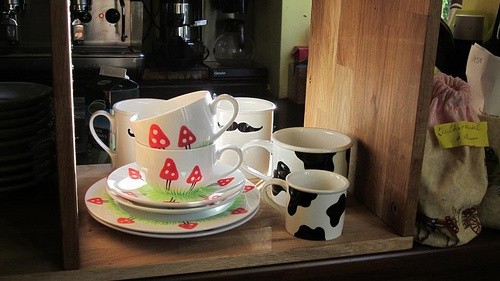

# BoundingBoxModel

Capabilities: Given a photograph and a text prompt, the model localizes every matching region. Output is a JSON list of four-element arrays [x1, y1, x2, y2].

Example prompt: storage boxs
[[288, 45, 309, 104]]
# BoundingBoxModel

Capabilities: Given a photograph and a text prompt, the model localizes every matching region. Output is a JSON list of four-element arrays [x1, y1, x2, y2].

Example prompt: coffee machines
[[130, 0, 210, 80]]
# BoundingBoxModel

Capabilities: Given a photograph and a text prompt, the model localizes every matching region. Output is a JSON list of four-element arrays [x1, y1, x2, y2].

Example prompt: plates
[[85, 176, 261, 238], [0, 81, 54, 191], [106, 162, 246, 216]]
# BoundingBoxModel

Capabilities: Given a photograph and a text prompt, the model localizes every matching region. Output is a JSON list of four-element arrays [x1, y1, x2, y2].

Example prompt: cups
[[261, 169, 350, 241], [135, 139, 243, 195], [240, 126, 353, 207], [213, 97, 277, 180], [89, 98, 166, 169], [129, 89, 239, 150]]
